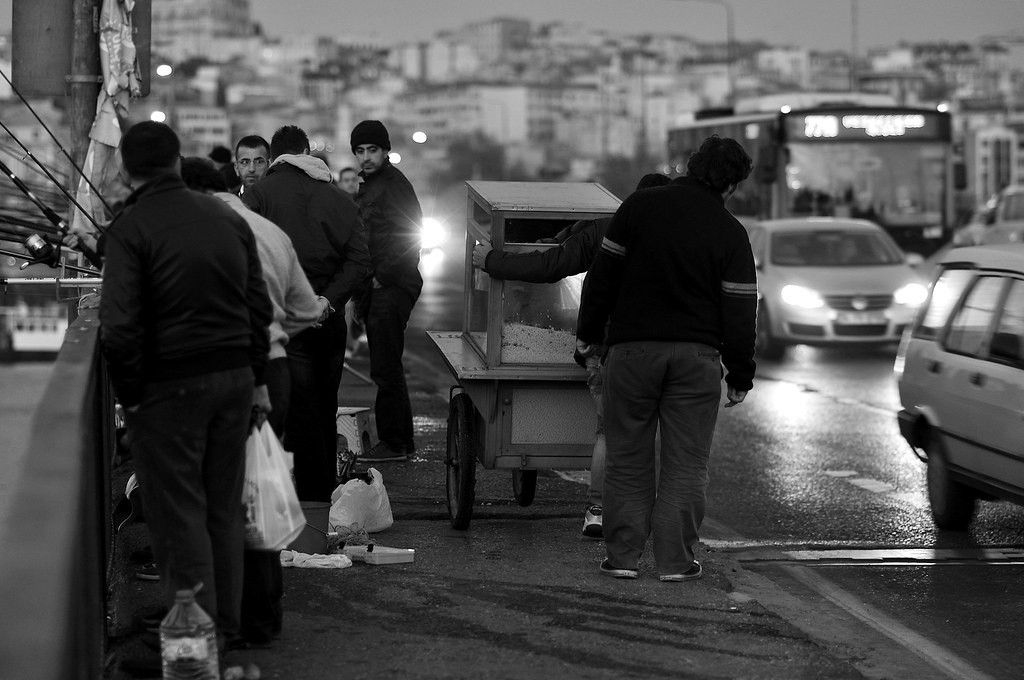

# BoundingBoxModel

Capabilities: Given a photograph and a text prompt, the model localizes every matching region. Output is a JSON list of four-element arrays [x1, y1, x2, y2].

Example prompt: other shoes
[[660, 559, 703, 582], [599, 557, 637, 578], [137, 561, 160, 579], [144, 608, 171, 623]]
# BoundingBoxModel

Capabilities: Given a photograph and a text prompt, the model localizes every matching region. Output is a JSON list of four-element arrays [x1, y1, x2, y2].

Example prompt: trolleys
[[425, 181, 599, 531]]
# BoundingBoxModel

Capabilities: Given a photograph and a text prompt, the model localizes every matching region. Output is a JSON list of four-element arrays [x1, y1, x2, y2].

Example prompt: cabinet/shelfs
[[462, 180, 624, 370]]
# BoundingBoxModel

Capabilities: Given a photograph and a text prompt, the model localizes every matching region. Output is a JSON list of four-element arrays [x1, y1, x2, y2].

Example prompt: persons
[[472, 173, 674, 537], [63, 229, 98, 256], [338, 167, 369, 363], [347, 120, 423, 462], [242, 125, 369, 505], [98, 121, 275, 649], [575, 133, 757, 583], [233, 135, 271, 197], [207, 146, 243, 195], [162, 155, 323, 644]]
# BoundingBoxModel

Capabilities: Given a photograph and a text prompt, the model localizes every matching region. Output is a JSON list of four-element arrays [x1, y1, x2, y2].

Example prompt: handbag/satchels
[[240, 419, 306, 552], [328, 466, 393, 535]]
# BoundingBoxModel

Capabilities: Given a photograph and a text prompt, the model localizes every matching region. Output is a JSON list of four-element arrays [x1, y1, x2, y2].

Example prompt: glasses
[[234, 157, 269, 167]]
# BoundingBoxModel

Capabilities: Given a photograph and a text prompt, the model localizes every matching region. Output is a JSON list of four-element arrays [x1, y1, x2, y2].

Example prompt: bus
[[667, 93, 967, 260]]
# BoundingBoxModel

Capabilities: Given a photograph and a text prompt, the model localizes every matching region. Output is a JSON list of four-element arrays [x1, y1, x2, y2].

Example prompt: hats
[[350, 120, 391, 152]]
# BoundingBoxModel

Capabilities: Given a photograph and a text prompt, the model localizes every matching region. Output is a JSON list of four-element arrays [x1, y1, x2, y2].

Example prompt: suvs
[[944, 184, 1024, 249]]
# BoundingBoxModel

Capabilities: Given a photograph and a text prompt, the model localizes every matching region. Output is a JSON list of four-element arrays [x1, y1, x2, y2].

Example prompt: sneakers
[[357, 438, 415, 461], [583, 505, 604, 538]]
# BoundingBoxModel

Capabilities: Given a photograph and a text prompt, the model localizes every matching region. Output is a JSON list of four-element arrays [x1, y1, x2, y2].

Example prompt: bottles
[[160, 582, 221, 680]]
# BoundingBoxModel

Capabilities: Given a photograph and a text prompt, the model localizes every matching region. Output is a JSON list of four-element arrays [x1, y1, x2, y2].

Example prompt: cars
[[746, 219, 926, 363], [893, 242, 1024, 534]]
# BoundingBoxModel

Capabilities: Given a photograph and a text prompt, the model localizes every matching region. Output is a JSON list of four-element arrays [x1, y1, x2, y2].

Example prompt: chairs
[[773, 242, 804, 265]]
